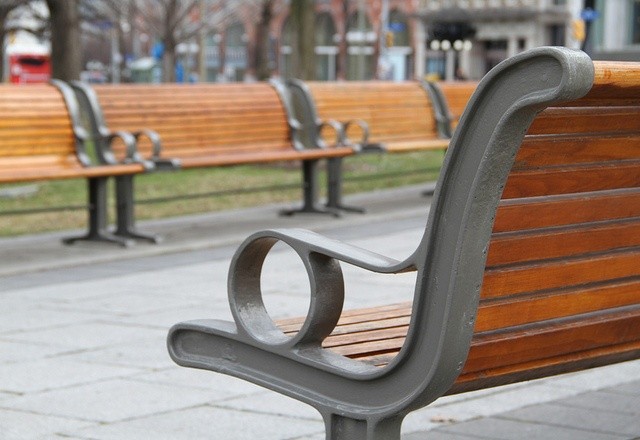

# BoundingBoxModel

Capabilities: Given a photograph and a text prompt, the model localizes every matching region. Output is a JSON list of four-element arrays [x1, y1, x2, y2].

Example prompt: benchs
[[1, 79, 154, 248], [166, 45, 640, 440], [67, 79, 360, 243], [289, 81, 460, 215], [432, 79, 479, 139]]
